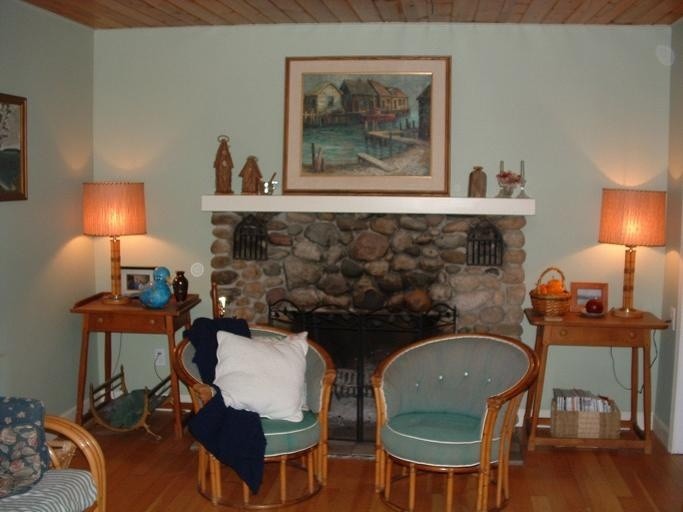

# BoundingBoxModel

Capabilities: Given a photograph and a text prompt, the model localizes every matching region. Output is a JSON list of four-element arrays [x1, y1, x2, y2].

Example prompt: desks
[[70, 291, 202, 439], [522, 306, 668, 453]]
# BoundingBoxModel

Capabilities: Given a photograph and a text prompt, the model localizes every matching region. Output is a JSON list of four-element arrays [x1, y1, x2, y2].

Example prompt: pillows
[[212, 330, 309, 423], [0, 397, 48, 499]]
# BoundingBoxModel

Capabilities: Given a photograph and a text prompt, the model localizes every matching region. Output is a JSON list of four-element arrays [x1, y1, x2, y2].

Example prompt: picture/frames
[[282, 57, 452, 198], [570, 282, 608, 313], [0, 93, 29, 202]]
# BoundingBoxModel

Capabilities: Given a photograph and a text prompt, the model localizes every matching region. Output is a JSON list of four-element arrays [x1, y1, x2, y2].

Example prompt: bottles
[[171, 270, 189, 302], [468, 166, 486, 198]]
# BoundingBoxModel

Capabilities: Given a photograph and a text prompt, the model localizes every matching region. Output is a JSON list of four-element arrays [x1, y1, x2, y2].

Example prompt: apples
[[586, 299, 603, 313]]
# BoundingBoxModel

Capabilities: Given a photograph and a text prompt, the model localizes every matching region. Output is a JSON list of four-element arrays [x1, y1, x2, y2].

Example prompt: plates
[[581, 309, 603, 317]]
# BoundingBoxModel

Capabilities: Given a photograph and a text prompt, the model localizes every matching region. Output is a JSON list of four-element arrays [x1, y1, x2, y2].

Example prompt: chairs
[[370, 332, 540, 512], [0, 414, 107, 512], [172, 319, 337, 511]]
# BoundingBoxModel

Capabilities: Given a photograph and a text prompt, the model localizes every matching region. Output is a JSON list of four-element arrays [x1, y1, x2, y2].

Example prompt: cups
[[257, 181, 277, 195]]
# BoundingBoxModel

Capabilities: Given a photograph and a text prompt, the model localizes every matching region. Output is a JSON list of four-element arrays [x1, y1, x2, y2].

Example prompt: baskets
[[530, 266, 571, 313]]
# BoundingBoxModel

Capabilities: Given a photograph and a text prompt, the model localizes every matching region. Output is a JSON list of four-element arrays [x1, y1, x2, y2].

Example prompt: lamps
[[82, 182, 147, 306], [598, 188, 666, 318]]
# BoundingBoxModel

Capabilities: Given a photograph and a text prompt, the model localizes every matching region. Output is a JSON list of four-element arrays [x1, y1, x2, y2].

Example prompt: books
[[553, 388, 612, 413]]
